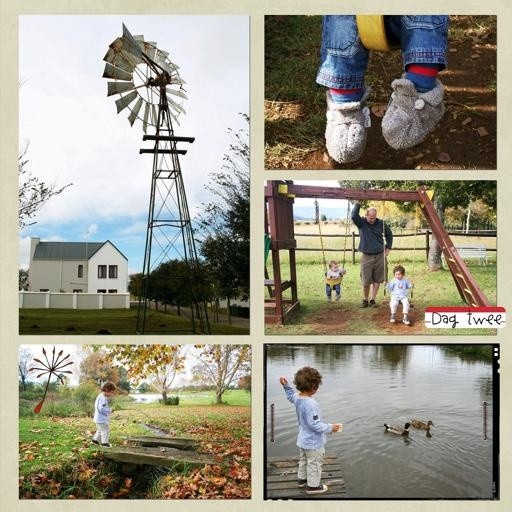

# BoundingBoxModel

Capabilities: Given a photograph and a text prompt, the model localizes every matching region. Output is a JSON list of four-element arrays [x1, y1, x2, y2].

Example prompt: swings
[[313, 197, 350, 289], [381, 200, 421, 308]]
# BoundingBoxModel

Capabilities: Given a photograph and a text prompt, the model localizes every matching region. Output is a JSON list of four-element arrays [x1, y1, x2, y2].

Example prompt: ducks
[[409, 417, 435, 430], [383, 422, 411, 436]]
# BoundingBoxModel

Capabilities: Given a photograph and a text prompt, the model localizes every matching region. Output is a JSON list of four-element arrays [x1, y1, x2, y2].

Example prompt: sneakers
[[402, 317, 411, 324], [324, 88, 373, 165], [327, 295, 341, 303], [91, 439, 99, 445], [100, 443, 112, 448], [305, 484, 328, 494], [297, 479, 307, 487], [380, 76, 446, 152], [360, 300, 378, 308], [390, 315, 397, 323]]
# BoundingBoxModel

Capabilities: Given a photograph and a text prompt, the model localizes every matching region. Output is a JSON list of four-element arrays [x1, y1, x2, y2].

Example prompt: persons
[[382, 265, 414, 325], [278, 365, 342, 494], [91, 381, 118, 448], [350, 198, 393, 308], [314, 15, 450, 164], [325, 259, 346, 304]]
